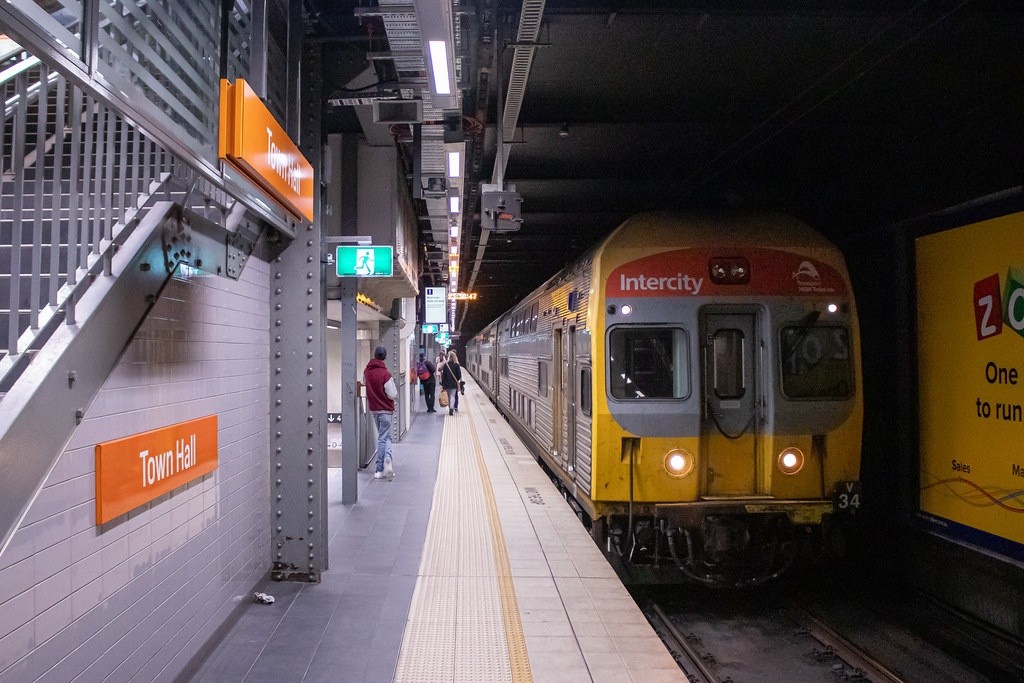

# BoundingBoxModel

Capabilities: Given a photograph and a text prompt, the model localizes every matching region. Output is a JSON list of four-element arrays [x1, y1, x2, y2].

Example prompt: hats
[[375, 346, 387, 355], [420, 354, 425, 357]]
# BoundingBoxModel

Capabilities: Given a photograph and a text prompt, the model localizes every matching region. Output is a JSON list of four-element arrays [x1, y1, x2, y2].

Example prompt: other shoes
[[455, 407, 458, 411], [449, 409, 453, 416], [426, 409, 437, 413]]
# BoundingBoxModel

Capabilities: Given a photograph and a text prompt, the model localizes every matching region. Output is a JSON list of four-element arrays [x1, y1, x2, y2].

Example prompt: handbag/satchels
[[457, 381, 461, 392], [439, 390, 448, 407]]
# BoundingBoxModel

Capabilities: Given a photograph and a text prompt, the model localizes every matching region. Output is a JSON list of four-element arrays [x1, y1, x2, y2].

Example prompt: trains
[[465, 199, 864, 589]]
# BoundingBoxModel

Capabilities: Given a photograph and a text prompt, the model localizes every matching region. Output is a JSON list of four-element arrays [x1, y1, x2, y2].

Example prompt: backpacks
[[417, 359, 430, 379]]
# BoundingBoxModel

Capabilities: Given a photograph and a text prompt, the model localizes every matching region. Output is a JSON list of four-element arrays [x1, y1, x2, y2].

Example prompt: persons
[[363, 345, 397, 481], [436, 349, 462, 415], [417, 353, 437, 413]]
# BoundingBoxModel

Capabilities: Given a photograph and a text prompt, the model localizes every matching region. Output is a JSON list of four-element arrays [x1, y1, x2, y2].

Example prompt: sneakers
[[374, 469, 396, 478], [384, 456, 394, 482]]
